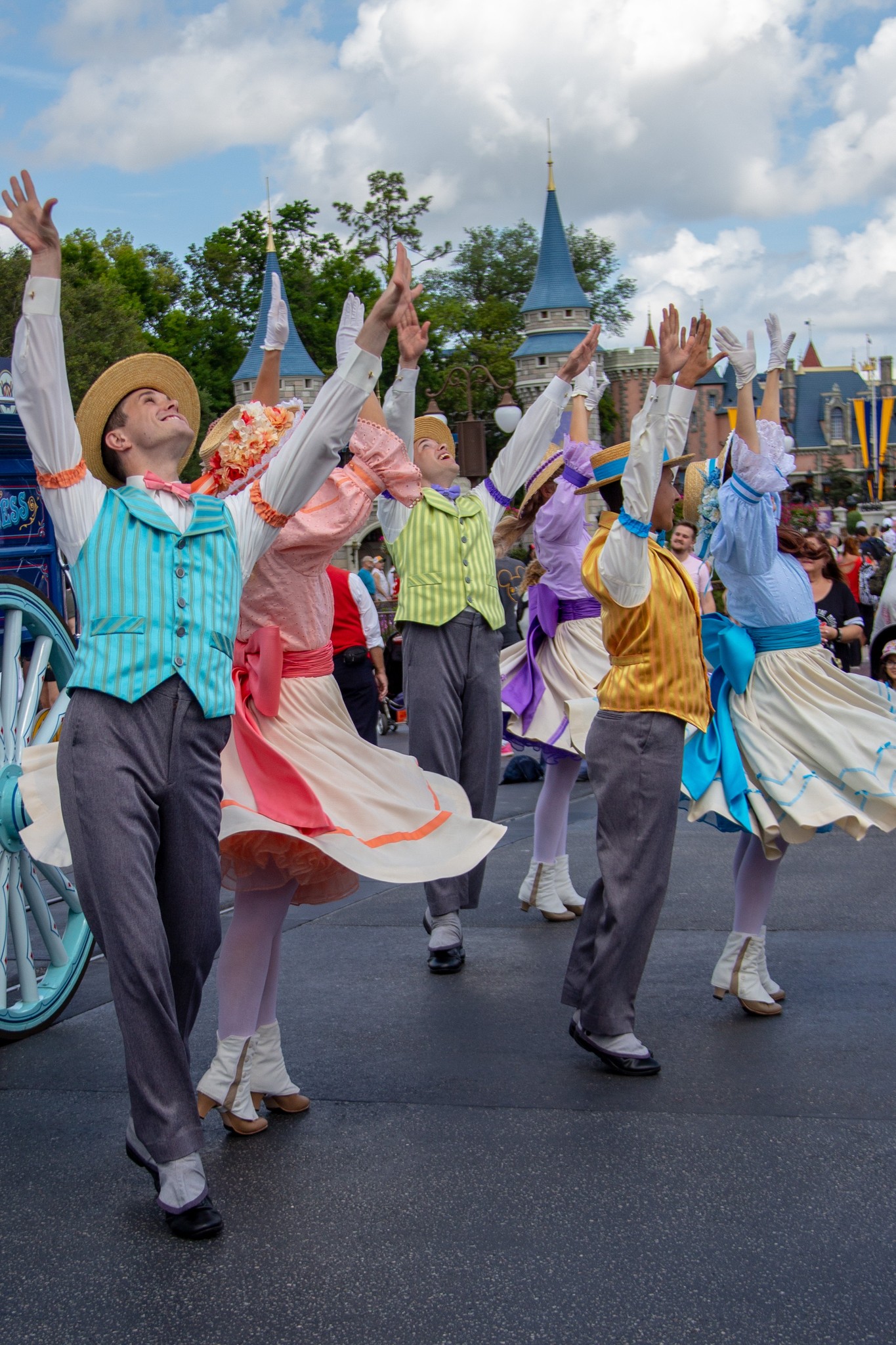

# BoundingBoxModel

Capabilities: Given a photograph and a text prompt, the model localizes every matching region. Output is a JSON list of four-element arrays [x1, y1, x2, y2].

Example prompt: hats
[[391, 569, 397, 573], [683, 429, 734, 535], [881, 640, 896, 658], [824, 528, 842, 547], [75, 353, 201, 489], [373, 555, 386, 563], [573, 440, 696, 497], [201, 397, 305, 500], [361, 556, 377, 561], [529, 544, 534, 549], [413, 416, 456, 460], [518, 442, 565, 520]]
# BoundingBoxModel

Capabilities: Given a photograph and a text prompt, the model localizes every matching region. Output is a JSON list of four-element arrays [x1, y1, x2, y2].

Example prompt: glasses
[[884, 659, 896, 668]]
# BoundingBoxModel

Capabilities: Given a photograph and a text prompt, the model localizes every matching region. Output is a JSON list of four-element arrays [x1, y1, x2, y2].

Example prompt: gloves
[[712, 325, 759, 390], [260, 273, 289, 350], [570, 360, 593, 399], [585, 362, 611, 412], [335, 292, 365, 369], [763, 312, 796, 373]]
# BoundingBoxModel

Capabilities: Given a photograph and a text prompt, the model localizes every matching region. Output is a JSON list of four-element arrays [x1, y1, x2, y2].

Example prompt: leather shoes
[[125, 1136, 223, 1239], [422, 914, 433, 935], [567, 1017, 661, 1076], [427, 944, 466, 975]]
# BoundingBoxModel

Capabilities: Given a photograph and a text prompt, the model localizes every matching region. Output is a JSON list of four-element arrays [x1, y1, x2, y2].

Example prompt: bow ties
[[143, 470, 192, 501], [431, 484, 460, 499], [656, 529, 665, 548]]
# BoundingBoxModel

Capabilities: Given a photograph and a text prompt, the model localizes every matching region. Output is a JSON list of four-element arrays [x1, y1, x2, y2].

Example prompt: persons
[[0, 171, 422, 1239], [723, 518, 896, 690], [21, 587, 81, 710], [667, 520, 715, 618], [491, 516, 589, 782], [501, 360, 610, 922], [196, 271, 393, 1136], [560, 302, 725, 1074], [374, 301, 600, 975], [711, 319, 818, 1016], [325, 556, 399, 748]]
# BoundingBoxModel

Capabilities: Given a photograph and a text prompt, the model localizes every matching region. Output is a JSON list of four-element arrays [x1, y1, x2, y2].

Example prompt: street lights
[[424, 365, 522, 488]]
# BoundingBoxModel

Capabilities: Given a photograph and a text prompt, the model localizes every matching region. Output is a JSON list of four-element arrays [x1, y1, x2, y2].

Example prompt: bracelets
[[826, 628, 839, 642], [827, 627, 843, 645]]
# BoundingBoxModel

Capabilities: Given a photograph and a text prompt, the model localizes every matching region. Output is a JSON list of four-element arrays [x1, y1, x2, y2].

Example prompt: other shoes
[[500, 743, 514, 757]]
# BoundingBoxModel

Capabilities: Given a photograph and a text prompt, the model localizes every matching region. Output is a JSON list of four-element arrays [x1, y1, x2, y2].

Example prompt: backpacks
[[867, 539, 886, 560]]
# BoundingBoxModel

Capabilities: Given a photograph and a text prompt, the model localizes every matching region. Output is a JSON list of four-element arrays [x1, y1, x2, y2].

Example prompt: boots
[[711, 925, 783, 1015], [196, 1028, 269, 1134], [250, 1017, 309, 1112], [553, 854, 586, 915], [518, 857, 576, 920]]
